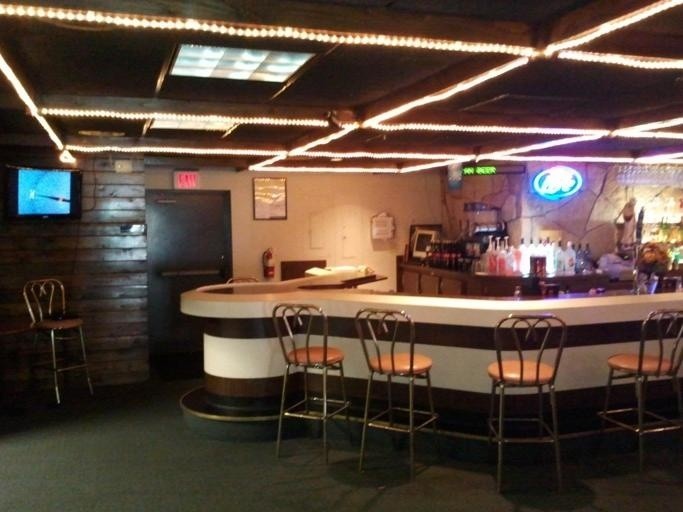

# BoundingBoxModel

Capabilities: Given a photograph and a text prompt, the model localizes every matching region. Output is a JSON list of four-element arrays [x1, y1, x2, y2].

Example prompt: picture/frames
[[252, 176, 287, 220]]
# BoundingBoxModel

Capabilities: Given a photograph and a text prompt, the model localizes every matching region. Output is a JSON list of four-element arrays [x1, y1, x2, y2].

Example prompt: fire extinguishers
[[262, 247, 275, 278]]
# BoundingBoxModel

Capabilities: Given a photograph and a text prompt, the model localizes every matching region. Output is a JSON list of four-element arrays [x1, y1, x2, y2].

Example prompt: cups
[[462, 202, 487, 210]]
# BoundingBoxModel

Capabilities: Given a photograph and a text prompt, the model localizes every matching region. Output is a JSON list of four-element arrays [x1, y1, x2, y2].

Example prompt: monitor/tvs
[[0, 165, 82, 224], [412, 229, 440, 258]]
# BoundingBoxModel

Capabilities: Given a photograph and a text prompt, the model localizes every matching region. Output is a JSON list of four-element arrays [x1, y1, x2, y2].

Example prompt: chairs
[[273, 302, 351, 465], [485, 311, 568, 496], [354, 307, 442, 480], [20, 278, 95, 404], [596, 307, 682, 477]]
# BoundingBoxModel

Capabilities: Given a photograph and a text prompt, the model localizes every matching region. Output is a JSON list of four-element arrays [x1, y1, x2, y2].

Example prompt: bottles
[[428, 233, 590, 274], [514, 285, 521, 301]]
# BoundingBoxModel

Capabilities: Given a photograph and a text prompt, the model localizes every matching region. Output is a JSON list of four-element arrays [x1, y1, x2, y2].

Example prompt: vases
[[640, 271, 658, 295]]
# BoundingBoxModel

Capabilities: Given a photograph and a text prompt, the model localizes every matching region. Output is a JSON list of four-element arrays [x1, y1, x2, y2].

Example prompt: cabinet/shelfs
[[398, 268, 638, 300]]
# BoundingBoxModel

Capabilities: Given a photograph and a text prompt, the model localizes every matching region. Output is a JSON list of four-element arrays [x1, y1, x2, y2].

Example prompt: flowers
[[634, 241, 672, 275]]
